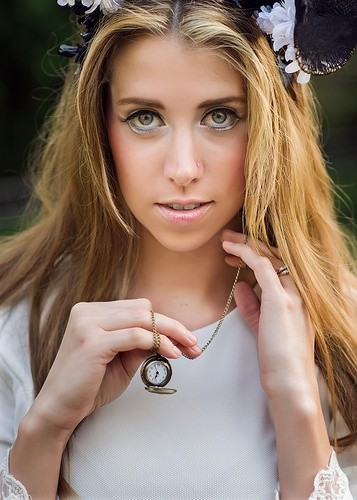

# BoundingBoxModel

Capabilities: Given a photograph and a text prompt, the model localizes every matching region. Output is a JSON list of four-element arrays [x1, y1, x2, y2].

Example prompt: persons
[[1, 1, 357, 500]]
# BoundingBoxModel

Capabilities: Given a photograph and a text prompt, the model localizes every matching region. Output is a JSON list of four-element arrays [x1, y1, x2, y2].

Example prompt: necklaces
[[140, 231, 249, 394]]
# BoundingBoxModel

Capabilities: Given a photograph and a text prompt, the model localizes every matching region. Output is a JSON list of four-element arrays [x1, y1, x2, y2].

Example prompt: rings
[[276, 265, 290, 276]]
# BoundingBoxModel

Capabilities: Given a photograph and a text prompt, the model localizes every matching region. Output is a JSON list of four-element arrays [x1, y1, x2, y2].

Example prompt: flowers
[[254, 0, 310, 83], [56, 0, 118, 16]]
